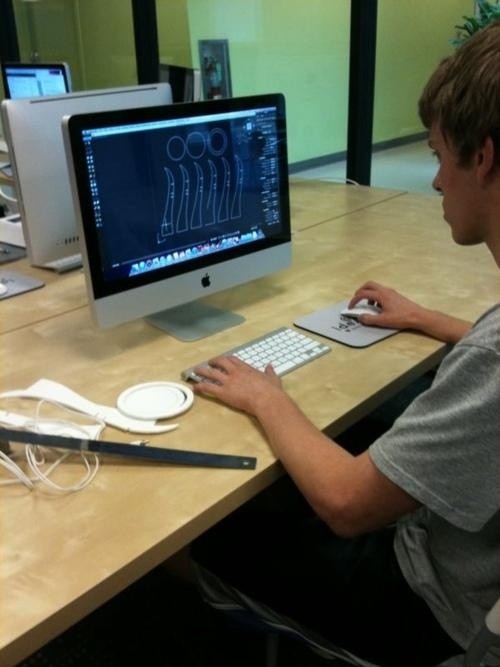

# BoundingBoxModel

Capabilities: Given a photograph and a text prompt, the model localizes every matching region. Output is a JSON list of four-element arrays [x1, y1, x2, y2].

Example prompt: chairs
[[255, 589, 500, 667]]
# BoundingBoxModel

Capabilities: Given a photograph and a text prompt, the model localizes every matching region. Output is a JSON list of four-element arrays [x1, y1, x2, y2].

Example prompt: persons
[[193, 14, 500, 667]]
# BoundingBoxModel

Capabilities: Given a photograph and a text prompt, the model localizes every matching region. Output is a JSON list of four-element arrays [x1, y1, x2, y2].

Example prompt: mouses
[[0, 282, 6, 296], [340, 304, 380, 320]]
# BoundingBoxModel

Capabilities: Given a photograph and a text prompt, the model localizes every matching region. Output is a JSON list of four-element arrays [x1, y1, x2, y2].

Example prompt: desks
[[1, 173, 407, 339], [1, 188, 500, 667]]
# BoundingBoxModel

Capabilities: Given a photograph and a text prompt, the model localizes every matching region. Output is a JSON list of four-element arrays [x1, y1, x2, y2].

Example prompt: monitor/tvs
[[0, 62, 72, 99], [0, 82, 173, 272], [61, 94, 292, 341]]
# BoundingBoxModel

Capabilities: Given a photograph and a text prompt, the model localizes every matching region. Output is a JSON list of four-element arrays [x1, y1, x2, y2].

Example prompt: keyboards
[[181, 328, 330, 395], [31, 253, 83, 274]]
